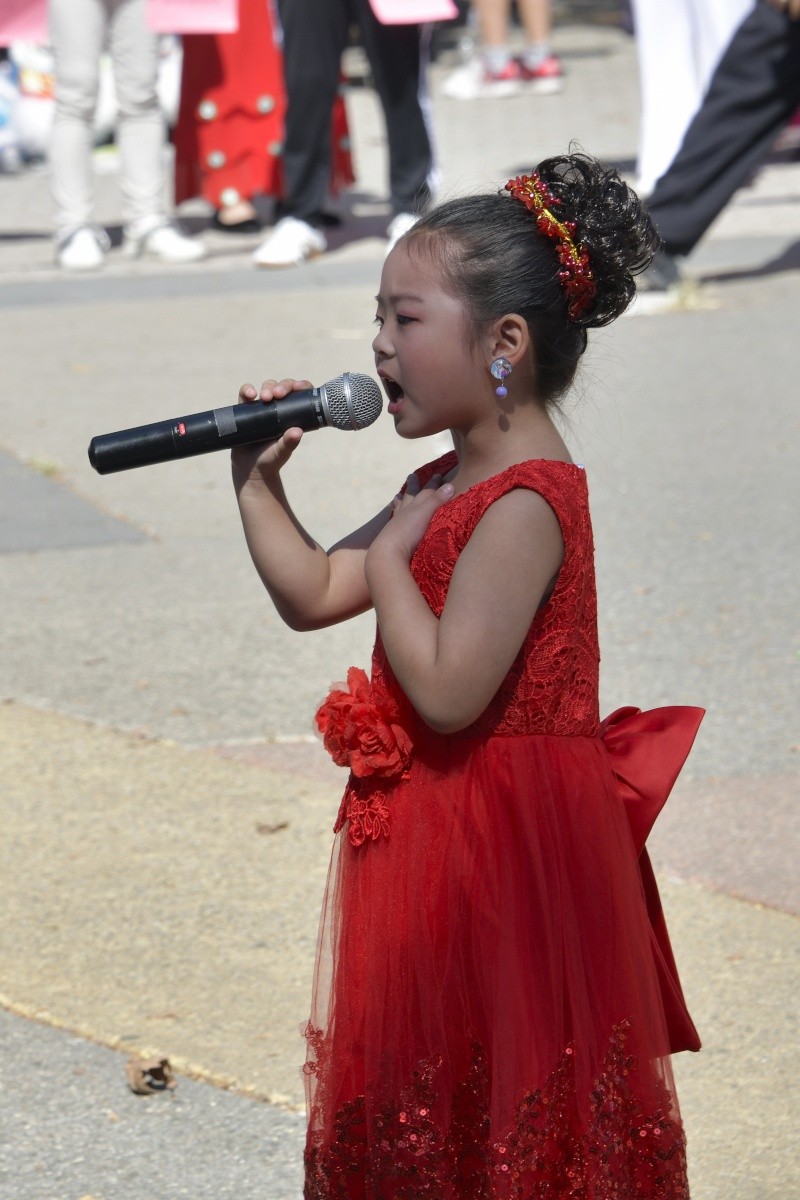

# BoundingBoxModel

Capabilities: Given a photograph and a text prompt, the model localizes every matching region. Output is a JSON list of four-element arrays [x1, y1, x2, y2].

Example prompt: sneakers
[[443, 60, 521, 98], [386, 214, 422, 256], [517, 55, 564, 94], [253, 216, 327, 266], [122, 227, 209, 262], [60, 229, 104, 269]]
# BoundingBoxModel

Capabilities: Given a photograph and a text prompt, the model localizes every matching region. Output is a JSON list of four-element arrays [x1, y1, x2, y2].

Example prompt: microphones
[[88, 371, 382, 475]]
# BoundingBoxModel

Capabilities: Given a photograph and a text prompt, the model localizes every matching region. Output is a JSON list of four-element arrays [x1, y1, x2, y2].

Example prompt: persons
[[229, 150, 707, 1200], [42, 1, 800, 290]]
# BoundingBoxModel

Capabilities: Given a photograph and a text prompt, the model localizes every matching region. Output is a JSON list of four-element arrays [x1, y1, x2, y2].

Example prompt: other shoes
[[643, 253, 679, 290]]
